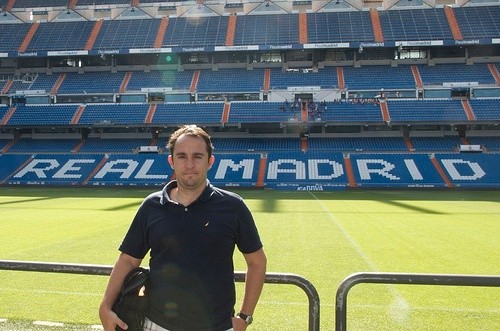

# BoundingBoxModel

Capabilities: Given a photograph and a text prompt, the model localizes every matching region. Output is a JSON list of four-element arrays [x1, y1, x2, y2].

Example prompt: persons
[[278, 96, 379, 122], [98, 124, 267, 331]]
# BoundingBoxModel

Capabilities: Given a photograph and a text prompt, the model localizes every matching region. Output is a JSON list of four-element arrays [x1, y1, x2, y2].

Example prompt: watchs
[[236, 312, 253, 325]]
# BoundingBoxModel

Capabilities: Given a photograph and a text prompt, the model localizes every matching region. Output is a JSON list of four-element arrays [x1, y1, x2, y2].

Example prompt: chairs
[[0, 0, 499, 192]]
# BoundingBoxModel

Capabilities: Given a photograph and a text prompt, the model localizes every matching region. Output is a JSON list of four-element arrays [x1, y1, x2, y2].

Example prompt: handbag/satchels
[[111, 267, 152, 330]]
